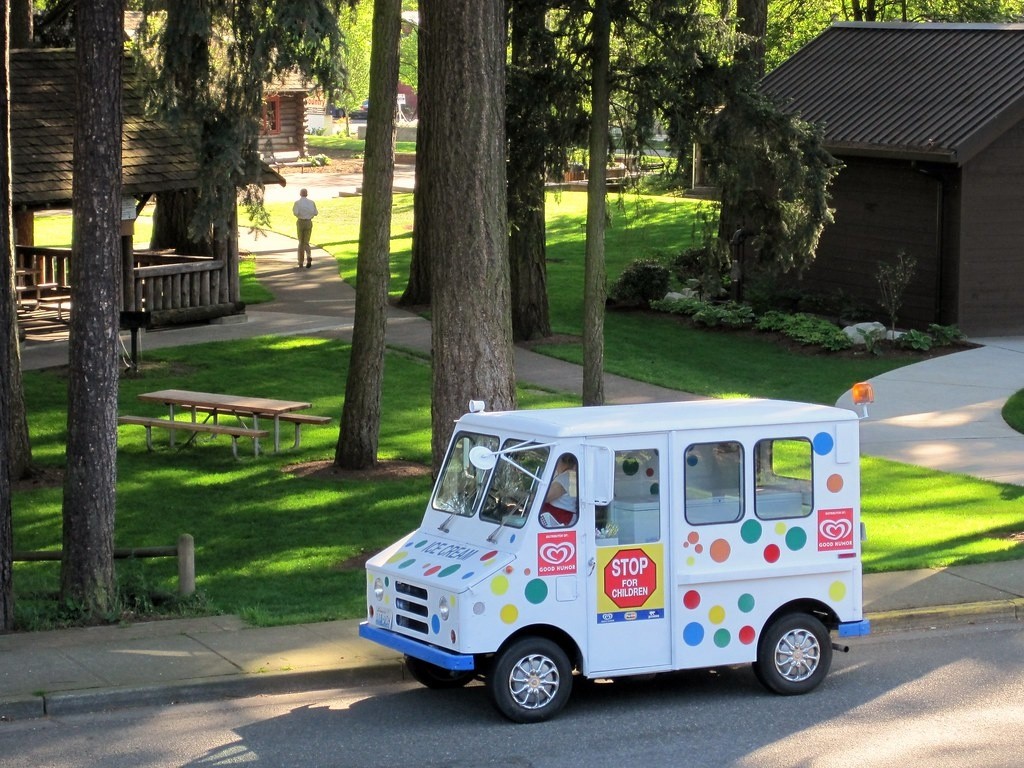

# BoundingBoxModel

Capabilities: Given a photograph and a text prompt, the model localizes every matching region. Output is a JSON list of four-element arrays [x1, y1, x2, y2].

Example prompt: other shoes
[[299, 264, 303, 268], [306, 258, 312, 268]]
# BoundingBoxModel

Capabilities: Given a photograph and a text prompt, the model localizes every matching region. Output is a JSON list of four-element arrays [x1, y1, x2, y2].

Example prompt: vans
[[358, 383, 874, 724]]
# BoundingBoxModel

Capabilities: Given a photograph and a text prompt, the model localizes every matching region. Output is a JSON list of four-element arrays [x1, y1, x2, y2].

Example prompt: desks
[[137, 390, 312, 459]]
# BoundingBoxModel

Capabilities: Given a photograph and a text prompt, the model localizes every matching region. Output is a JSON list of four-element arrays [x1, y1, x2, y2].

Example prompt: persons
[[495, 452, 577, 527], [293, 189, 318, 268]]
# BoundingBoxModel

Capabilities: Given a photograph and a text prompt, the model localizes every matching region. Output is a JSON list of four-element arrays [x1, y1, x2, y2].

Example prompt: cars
[[350, 100, 368, 119]]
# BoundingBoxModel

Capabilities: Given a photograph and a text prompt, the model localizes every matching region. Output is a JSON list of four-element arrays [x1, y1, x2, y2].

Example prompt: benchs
[[182, 404, 332, 450], [39, 295, 71, 321], [16, 268, 42, 291], [15, 283, 58, 308], [272, 151, 311, 175], [117, 415, 269, 461]]
